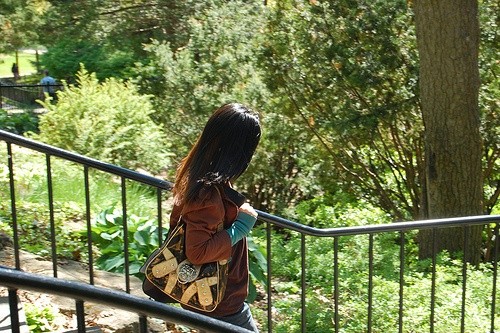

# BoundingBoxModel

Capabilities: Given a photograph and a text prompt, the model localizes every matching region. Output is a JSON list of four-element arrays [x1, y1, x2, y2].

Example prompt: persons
[[170, 101, 261, 333], [39, 71, 55, 99], [12, 63, 18, 81]]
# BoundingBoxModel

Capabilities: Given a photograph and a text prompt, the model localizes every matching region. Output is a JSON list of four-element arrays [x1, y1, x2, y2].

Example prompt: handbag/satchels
[[139, 179, 246, 313]]
